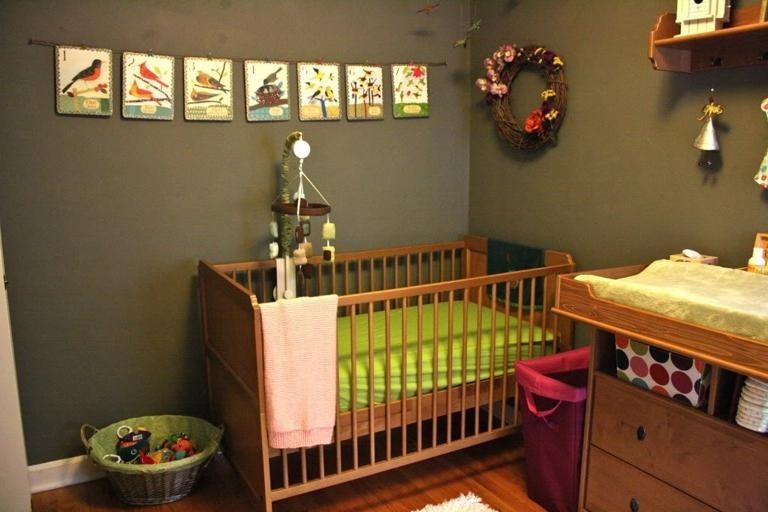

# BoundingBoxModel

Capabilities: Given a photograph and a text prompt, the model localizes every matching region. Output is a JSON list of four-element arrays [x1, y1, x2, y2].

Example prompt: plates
[[735, 375, 768, 434]]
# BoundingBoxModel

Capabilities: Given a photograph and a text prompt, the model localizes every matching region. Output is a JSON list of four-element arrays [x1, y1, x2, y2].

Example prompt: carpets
[[410, 491, 499, 512]]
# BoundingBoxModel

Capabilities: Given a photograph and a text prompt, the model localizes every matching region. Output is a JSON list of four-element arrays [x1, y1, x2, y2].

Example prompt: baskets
[[80, 415, 226, 507]]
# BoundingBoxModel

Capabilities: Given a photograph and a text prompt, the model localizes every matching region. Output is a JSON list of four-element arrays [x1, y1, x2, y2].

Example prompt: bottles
[[748, 247, 765, 268]]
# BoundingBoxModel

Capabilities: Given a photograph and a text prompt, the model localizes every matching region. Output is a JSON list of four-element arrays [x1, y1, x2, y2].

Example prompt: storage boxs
[[514, 344, 592, 512], [615, 333, 711, 409]]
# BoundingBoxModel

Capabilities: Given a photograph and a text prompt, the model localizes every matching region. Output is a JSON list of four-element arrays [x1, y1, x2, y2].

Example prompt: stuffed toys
[[103, 425, 202, 464]]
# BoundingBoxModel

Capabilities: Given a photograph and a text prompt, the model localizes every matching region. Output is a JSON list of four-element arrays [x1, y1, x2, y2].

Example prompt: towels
[[258, 293, 339, 449]]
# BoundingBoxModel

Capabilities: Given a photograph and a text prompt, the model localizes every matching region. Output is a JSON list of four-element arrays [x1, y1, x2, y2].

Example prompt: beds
[[199, 234, 575, 512]]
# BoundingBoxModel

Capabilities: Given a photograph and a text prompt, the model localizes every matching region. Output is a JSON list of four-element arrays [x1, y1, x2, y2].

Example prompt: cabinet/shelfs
[[551, 258, 768, 512]]
